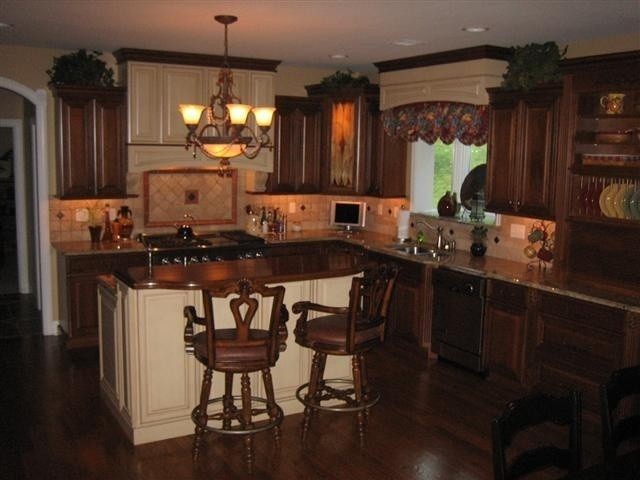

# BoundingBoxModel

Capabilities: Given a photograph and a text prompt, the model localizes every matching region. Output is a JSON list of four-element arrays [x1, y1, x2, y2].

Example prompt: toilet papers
[[396, 208, 410, 240]]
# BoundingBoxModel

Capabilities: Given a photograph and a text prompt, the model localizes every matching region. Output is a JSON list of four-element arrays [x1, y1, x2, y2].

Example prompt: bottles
[[244, 203, 287, 235], [102, 202, 135, 241]]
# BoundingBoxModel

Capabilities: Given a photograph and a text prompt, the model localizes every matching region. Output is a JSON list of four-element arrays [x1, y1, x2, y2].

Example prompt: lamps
[[178, 15, 277, 177]]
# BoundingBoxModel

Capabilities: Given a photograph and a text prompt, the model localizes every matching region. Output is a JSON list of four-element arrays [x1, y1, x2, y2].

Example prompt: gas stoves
[[137, 230, 261, 250]]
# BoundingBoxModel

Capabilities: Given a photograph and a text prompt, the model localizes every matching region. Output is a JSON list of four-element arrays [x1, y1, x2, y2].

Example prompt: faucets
[[409, 218, 455, 250]]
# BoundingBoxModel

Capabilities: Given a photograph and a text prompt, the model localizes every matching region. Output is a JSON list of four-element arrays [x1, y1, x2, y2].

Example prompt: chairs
[[184, 278, 288, 474], [600, 365, 640, 462], [490, 391, 583, 480], [293, 262, 399, 448]]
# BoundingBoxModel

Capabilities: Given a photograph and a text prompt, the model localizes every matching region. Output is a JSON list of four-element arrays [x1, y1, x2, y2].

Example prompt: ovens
[[427, 267, 490, 374]]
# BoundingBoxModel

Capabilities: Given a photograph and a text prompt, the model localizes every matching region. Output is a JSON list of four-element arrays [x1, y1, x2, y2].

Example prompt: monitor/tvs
[[329, 200, 367, 234]]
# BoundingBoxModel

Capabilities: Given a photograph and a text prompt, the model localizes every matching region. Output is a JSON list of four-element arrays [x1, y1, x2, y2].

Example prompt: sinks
[[395, 244, 432, 254], [416, 251, 453, 261]]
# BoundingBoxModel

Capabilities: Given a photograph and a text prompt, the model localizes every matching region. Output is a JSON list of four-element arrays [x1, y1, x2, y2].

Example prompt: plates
[[574, 181, 640, 222]]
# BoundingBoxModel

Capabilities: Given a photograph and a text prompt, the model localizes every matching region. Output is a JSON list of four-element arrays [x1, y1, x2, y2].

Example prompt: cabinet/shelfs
[[53, 85, 127, 200], [112, 47, 280, 175], [484, 282, 640, 425], [268, 83, 406, 200], [365, 255, 434, 362], [555, 50, 640, 293], [484, 85, 562, 220]]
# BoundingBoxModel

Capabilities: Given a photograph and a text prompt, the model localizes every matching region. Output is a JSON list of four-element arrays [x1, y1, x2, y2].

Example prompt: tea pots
[[173, 214, 195, 238], [598, 92, 626, 117]]
